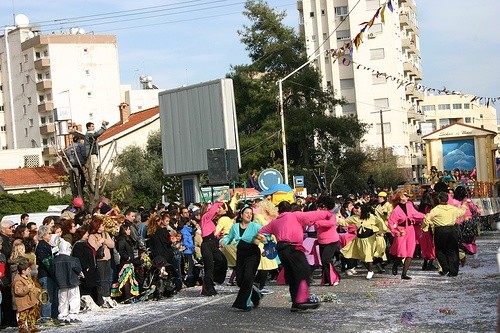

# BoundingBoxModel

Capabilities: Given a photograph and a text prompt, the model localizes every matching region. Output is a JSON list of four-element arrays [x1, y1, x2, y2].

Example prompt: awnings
[[260, 184, 294, 195]]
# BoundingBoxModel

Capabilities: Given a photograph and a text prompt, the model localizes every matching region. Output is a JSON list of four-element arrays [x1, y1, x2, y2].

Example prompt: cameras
[[106, 122, 109, 125]]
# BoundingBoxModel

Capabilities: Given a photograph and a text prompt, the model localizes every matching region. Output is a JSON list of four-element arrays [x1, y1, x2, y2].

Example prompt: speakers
[[207, 148, 240, 186]]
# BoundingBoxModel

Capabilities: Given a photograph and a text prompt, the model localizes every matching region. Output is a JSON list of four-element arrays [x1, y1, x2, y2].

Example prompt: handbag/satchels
[[95, 247, 105, 259]]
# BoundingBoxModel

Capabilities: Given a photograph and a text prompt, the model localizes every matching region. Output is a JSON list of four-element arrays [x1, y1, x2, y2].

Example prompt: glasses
[[9, 226, 13, 229]]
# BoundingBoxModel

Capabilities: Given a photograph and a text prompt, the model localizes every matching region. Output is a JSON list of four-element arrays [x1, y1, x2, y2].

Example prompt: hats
[[430, 166, 437, 170], [9, 221, 18, 226], [378, 191, 387, 197]]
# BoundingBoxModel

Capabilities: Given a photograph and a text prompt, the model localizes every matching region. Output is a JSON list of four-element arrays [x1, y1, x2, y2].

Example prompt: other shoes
[[259, 289, 275, 295], [421, 264, 438, 271], [324, 282, 339, 286], [401, 275, 412, 279], [461, 257, 466, 267], [235, 306, 256, 311], [345, 269, 357, 275], [366, 271, 375, 279], [392, 266, 397, 274], [60, 319, 82, 325], [292, 302, 321, 311], [19, 328, 40, 333]]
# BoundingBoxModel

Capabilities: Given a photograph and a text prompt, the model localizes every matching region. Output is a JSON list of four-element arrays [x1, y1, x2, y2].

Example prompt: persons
[[254, 201, 334, 312], [0, 200, 235, 333], [65, 136, 87, 199], [422, 191, 467, 277], [388, 189, 426, 280], [218, 204, 274, 312], [236, 192, 397, 294], [85, 121, 109, 189], [404, 166, 481, 276]]
